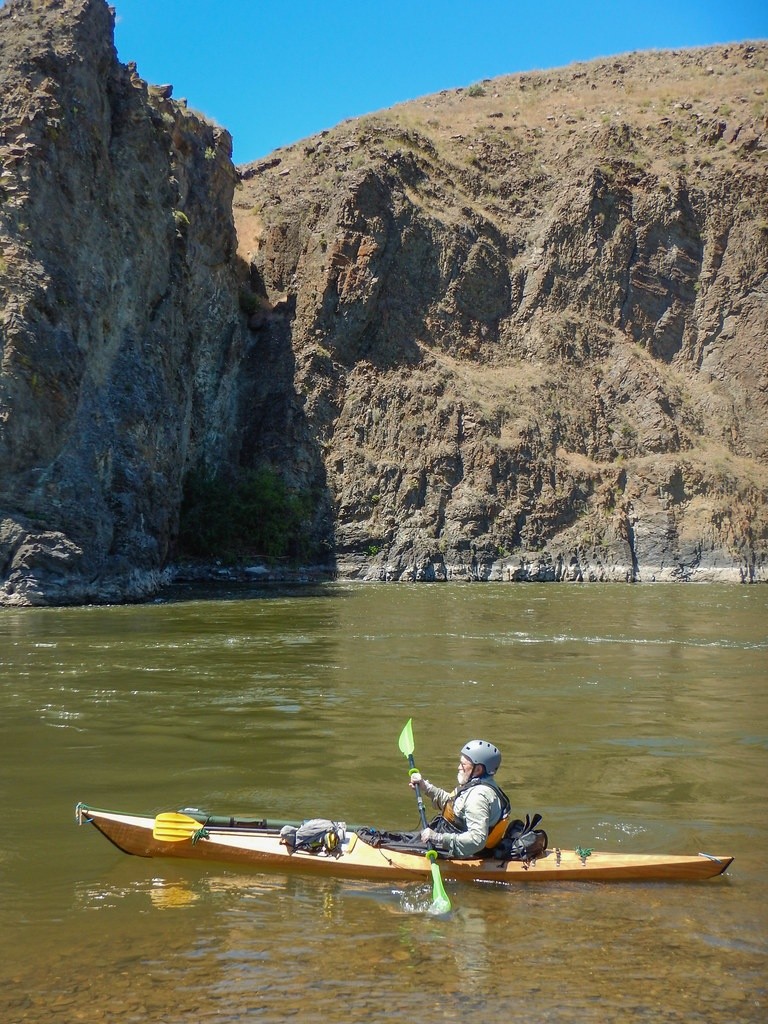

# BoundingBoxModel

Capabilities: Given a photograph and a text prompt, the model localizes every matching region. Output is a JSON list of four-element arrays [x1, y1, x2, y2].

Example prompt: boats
[[75, 802, 734, 885]]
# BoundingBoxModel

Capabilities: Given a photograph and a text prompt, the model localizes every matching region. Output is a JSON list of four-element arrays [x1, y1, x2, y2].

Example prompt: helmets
[[461, 740, 502, 775]]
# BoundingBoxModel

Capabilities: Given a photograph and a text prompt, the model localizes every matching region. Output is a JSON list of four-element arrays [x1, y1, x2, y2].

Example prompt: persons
[[408, 740, 512, 857]]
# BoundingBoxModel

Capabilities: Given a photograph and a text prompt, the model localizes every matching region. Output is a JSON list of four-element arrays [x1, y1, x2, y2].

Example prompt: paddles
[[396, 716, 453, 910]]
